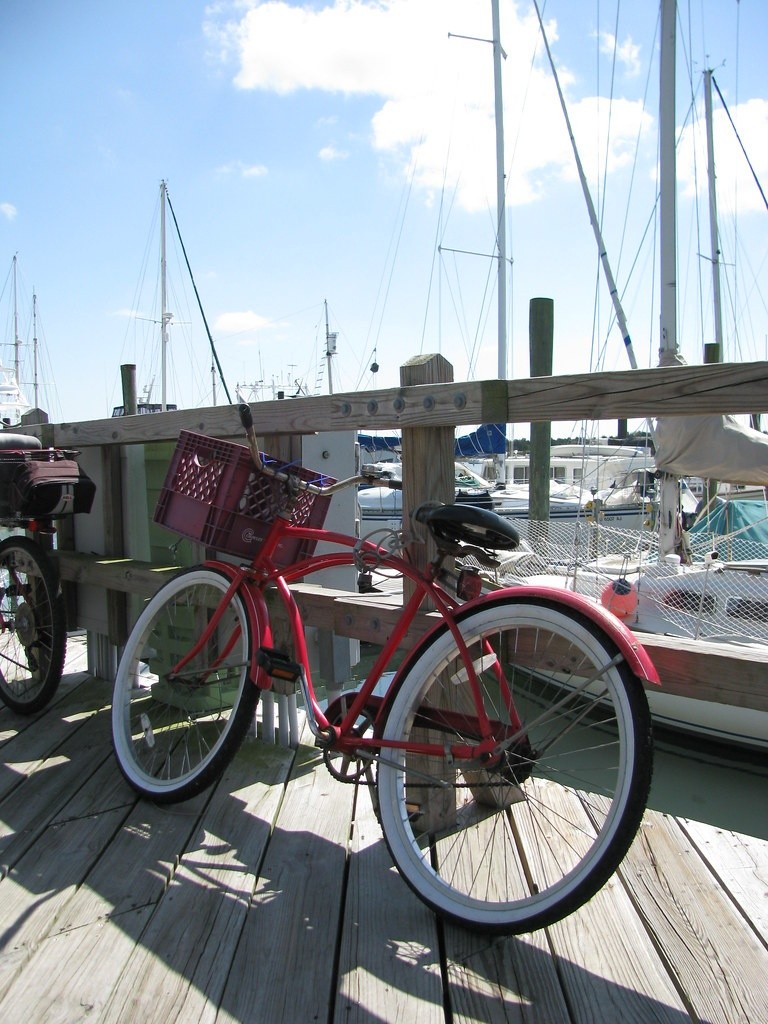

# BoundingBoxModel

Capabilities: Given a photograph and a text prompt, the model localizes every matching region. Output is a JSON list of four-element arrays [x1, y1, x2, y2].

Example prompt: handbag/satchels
[[8, 460, 96, 516]]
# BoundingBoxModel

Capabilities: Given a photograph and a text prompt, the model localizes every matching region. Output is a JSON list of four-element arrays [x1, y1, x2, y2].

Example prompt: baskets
[[153, 430, 339, 571]]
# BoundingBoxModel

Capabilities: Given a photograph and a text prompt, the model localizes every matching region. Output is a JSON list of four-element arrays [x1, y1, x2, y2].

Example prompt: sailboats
[[0, 1, 767, 777]]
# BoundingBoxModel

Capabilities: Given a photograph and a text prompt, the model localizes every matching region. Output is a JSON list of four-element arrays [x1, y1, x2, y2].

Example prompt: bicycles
[[0, 433, 75, 717], [109, 404, 663, 938]]
